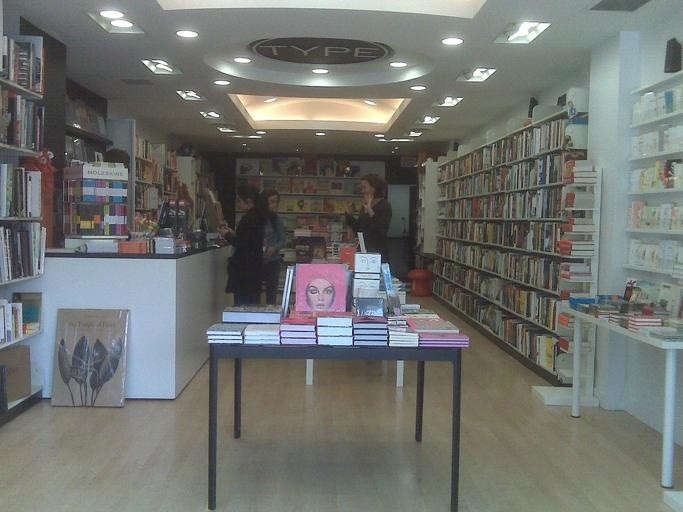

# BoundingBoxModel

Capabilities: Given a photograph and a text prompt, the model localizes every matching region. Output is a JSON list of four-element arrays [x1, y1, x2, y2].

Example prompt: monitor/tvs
[[157, 201, 168, 225]]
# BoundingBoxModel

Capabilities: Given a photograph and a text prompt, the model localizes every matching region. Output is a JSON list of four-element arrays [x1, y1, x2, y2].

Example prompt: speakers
[[453, 141, 459, 151], [527, 96, 538, 118], [663, 38, 681, 73]]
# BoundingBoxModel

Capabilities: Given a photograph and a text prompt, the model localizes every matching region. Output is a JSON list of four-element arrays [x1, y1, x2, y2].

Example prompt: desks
[[208, 347, 463, 512], [560, 304, 682, 487]]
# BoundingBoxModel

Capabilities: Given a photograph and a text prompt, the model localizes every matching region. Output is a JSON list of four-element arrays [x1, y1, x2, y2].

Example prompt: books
[[432, 118, 594, 373], [64, 234, 188, 256], [134, 134, 194, 233], [235, 158, 361, 268], [570, 86, 683, 342], [207, 253, 469, 346], [66, 93, 107, 166], [2, 32, 48, 347]]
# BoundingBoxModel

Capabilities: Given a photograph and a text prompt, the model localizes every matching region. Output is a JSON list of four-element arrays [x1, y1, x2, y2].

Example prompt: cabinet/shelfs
[[2, 78, 215, 426], [412, 87, 598, 390], [622, 70, 683, 278], [234, 158, 365, 233]]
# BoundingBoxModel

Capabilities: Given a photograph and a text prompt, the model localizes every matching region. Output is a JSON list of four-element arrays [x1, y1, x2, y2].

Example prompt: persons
[[344, 174, 393, 263], [218, 183, 286, 307]]
